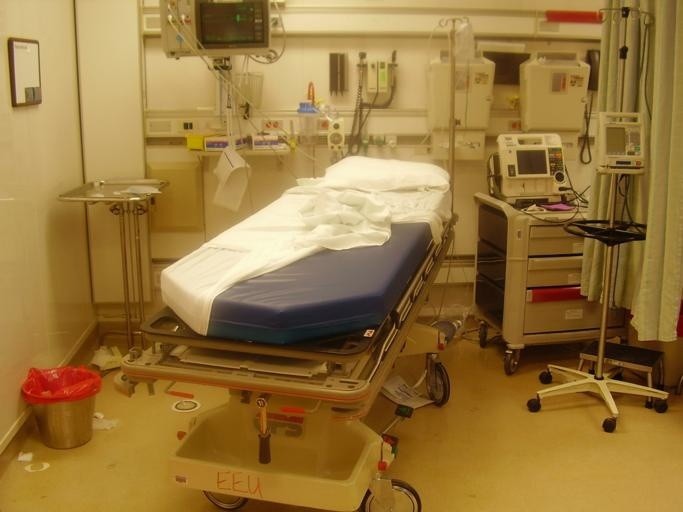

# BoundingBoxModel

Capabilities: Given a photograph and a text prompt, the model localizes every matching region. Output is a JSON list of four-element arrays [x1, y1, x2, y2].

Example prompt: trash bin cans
[[21, 367, 99, 450]]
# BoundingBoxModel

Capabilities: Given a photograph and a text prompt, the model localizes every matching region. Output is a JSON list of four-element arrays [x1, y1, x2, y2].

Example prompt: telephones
[[587, 49, 600, 90]]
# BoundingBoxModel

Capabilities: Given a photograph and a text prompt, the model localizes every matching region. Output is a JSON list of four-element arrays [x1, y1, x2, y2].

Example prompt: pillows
[[322, 153, 449, 194]]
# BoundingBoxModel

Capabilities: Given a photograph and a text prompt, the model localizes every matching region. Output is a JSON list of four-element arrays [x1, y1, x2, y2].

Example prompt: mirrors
[[10, 38, 42, 108]]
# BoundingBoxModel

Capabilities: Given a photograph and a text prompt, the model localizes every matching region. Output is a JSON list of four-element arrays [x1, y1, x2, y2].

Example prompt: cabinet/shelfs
[[470, 192, 629, 377]]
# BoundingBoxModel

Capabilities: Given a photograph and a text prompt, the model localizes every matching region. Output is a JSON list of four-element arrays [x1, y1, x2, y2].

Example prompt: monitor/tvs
[[196, 0, 268, 49]]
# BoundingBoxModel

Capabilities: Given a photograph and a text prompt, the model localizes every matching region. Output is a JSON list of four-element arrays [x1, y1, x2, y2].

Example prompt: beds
[[158, 177, 451, 348]]
